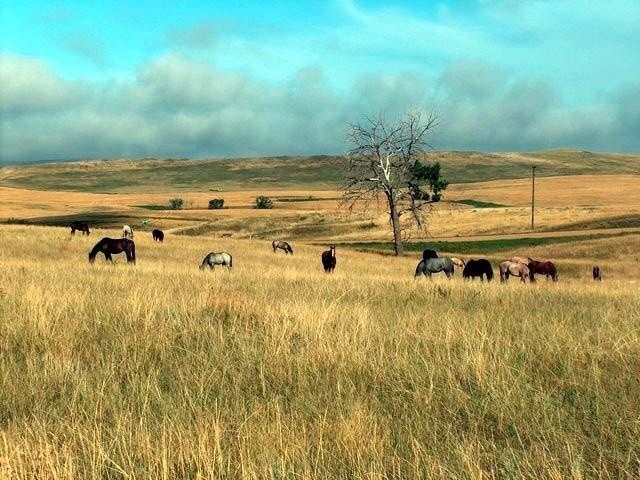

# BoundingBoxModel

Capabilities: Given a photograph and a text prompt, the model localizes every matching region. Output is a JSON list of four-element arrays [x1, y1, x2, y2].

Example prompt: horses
[[88, 236, 136, 265], [122, 224, 134, 238], [321, 244, 337, 273], [198, 251, 233, 271], [413, 248, 559, 283], [272, 239, 294, 255], [592, 265, 602, 281], [70, 222, 91, 236], [152, 229, 165, 243]]
